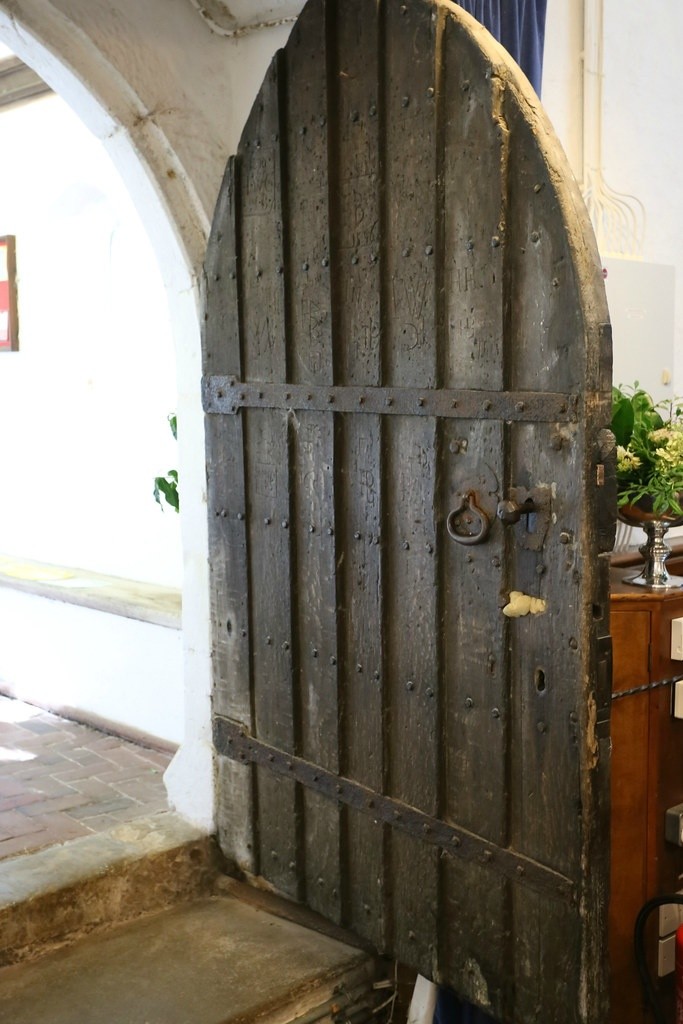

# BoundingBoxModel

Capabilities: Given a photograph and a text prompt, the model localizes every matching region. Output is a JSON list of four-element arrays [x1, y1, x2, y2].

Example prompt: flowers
[[611, 380, 683, 518]]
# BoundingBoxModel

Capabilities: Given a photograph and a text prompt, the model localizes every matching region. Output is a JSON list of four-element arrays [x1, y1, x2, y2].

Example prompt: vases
[[616, 501, 683, 589]]
